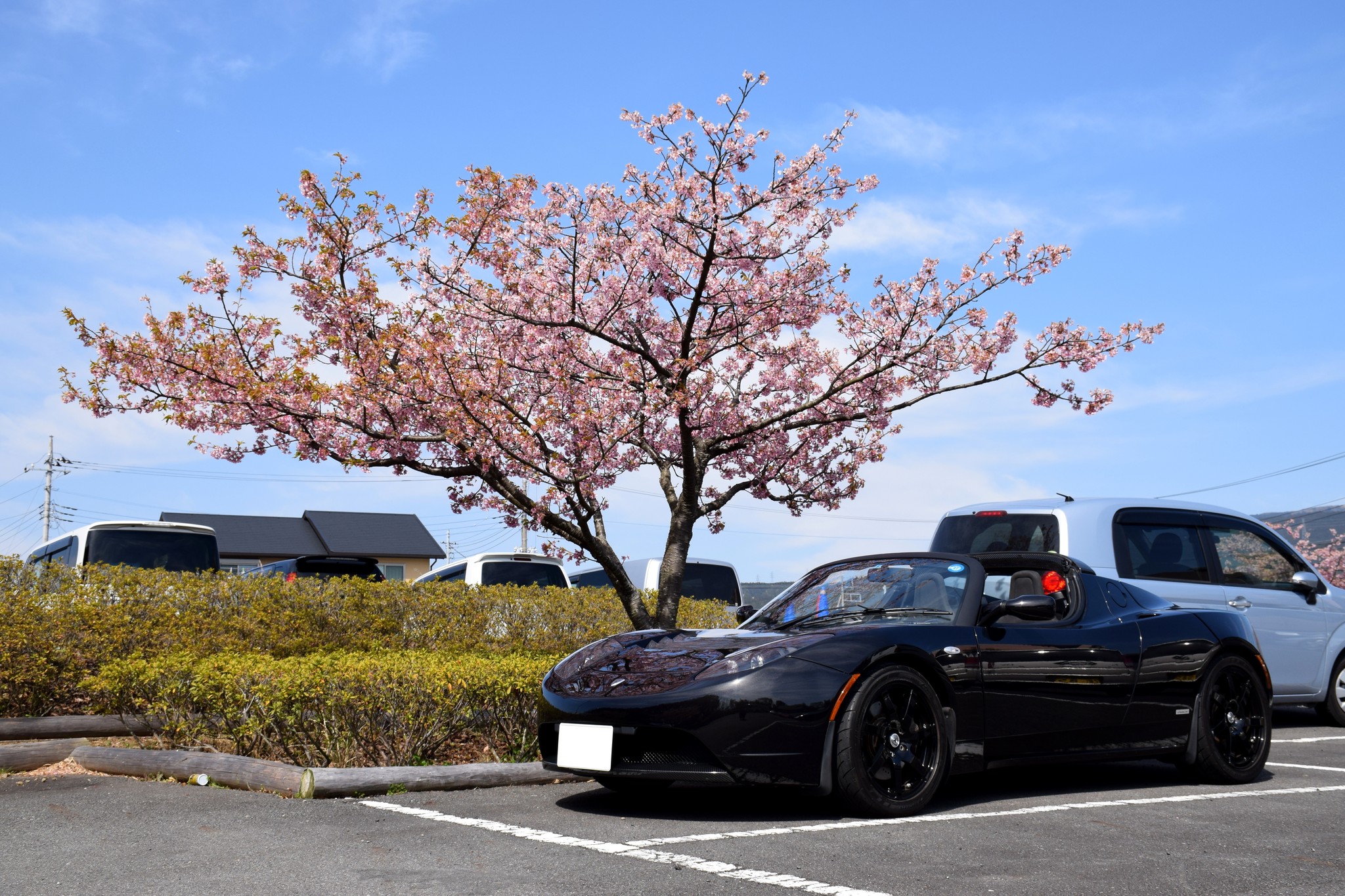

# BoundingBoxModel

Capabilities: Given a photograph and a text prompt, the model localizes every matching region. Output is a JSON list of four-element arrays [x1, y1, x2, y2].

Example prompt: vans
[[565, 555, 746, 626], [402, 552, 574, 640], [920, 495, 1345, 730], [16, 520, 220, 615]]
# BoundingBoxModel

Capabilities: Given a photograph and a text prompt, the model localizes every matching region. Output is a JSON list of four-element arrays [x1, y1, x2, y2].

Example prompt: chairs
[[997, 570, 1059, 624], [905, 572, 956, 621], [1149, 532, 1183, 578]]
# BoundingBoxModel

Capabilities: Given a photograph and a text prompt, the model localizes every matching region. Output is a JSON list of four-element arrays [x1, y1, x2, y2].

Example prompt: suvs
[[237, 555, 388, 586]]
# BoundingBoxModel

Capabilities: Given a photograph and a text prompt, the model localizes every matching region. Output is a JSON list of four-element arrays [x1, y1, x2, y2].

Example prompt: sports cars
[[540, 551, 1277, 819]]
